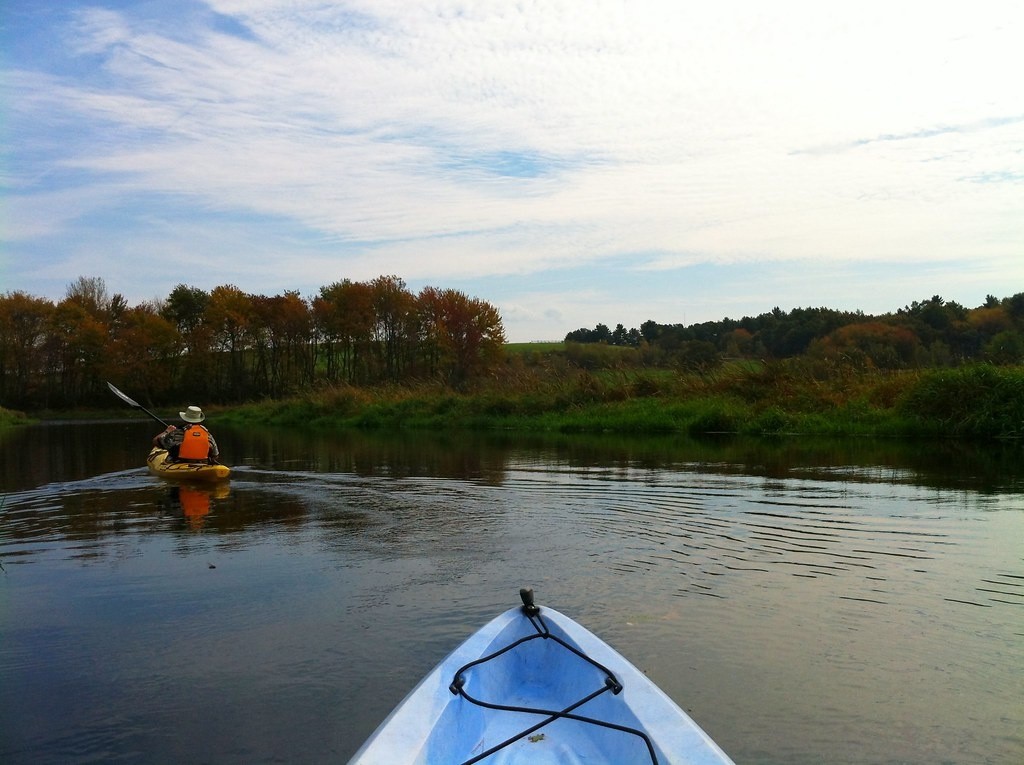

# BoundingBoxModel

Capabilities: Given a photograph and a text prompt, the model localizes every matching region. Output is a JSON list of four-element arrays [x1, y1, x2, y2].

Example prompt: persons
[[152, 406, 220, 463]]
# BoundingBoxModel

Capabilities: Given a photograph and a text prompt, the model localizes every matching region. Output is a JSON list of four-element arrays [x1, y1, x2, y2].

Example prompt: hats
[[179, 406, 205, 423]]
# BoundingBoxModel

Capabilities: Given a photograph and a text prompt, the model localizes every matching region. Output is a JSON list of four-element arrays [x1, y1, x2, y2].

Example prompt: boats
[[146, 445, 230, 484]]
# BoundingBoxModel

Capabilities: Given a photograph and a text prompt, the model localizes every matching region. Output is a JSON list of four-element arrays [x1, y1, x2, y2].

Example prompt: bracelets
[[165, 430, 168, 433]]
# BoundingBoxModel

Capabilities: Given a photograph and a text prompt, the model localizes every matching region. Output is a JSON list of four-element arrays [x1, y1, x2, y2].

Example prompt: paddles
[[107, 379, 168, 428]]
